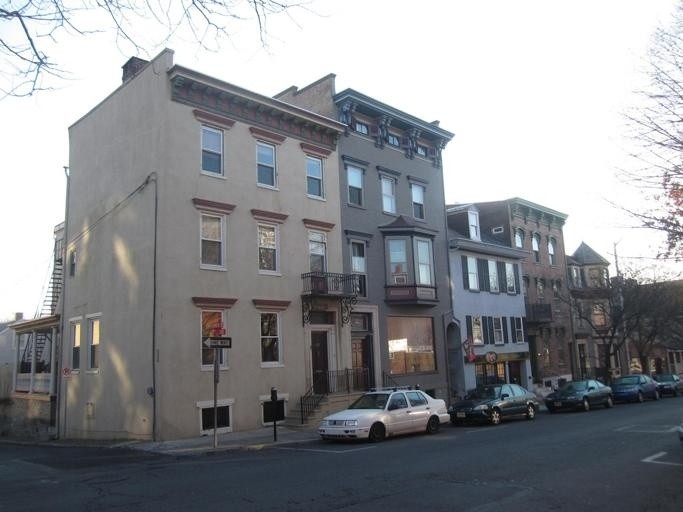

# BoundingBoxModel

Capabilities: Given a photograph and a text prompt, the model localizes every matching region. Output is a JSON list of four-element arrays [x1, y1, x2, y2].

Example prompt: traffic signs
[[202, 337, 232, 349]]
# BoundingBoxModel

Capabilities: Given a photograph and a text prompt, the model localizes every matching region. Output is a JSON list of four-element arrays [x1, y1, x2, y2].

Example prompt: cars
[[448, 384, 540, 427], [611, 375, 661, 404], [654, 375, 683, 396], [545, 379, 614, 415], [320, 390, 450, 442]]
